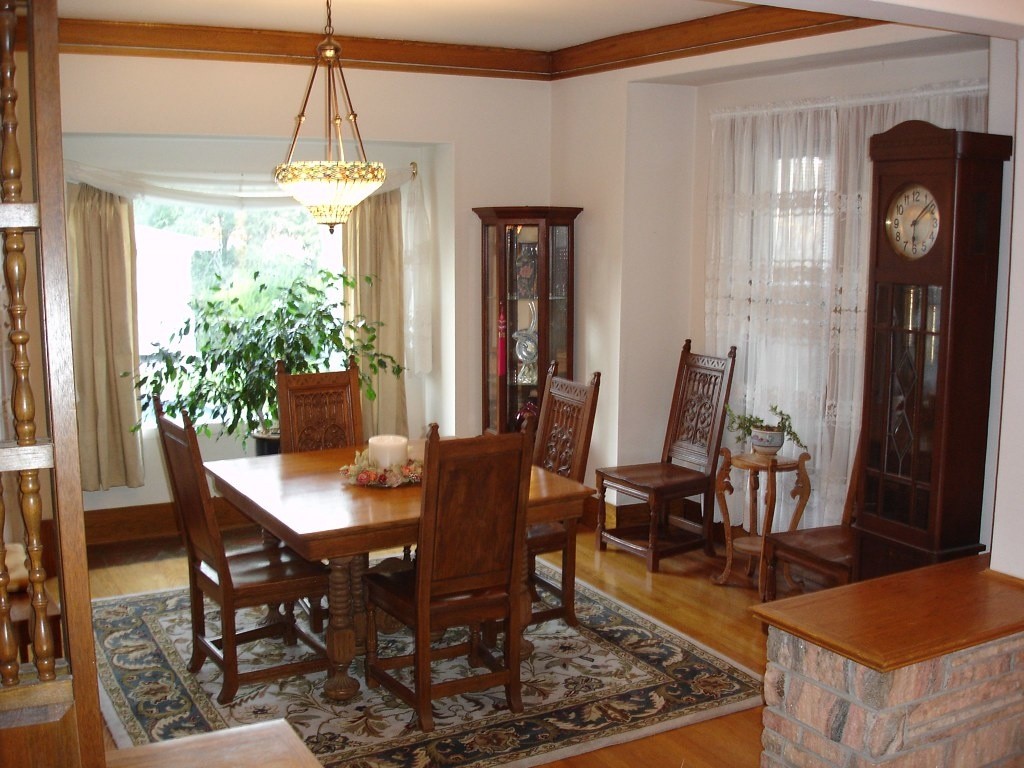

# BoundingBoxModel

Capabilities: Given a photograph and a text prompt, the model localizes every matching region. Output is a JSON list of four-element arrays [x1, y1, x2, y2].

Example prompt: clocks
[[850, 119, 1014, 575]]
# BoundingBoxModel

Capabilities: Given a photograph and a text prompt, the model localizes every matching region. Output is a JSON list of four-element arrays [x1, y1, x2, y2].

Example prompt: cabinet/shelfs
[[472, 206, 584, 435]]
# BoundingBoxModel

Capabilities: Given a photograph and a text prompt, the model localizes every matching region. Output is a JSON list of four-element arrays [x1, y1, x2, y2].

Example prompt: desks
[[203, 436, 597, 699]]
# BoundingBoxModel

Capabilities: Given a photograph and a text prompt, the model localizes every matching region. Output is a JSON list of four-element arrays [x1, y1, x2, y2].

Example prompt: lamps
[[277, 0, 386, 235]]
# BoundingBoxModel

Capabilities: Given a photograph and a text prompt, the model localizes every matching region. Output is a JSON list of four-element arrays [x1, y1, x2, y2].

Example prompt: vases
[[516, 242, 538, 299]]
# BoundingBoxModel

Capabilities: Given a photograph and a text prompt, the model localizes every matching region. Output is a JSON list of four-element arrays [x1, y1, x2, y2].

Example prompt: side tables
[[711, 447, 812, 603]]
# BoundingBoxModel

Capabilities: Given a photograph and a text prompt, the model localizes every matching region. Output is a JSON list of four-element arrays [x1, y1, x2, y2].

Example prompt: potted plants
[[725, 403, 808, 457], [120, 272, 406, 457]]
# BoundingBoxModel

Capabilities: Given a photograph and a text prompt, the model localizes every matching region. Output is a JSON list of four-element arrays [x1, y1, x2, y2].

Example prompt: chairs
[[757, 428, 854, 635], [153, 397, 332, 704], [277, 355, 364, 453], [532, 362, 602, 626], [596, 339, 737, 571], [362, 413, 537, 732]]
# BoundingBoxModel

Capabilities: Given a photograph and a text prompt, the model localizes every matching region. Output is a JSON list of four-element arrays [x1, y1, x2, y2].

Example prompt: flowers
[[340, 459, 423, 484]]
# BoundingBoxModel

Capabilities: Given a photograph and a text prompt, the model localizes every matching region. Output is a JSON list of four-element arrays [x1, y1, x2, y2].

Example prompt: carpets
[[91, 538, 766, 768]]
